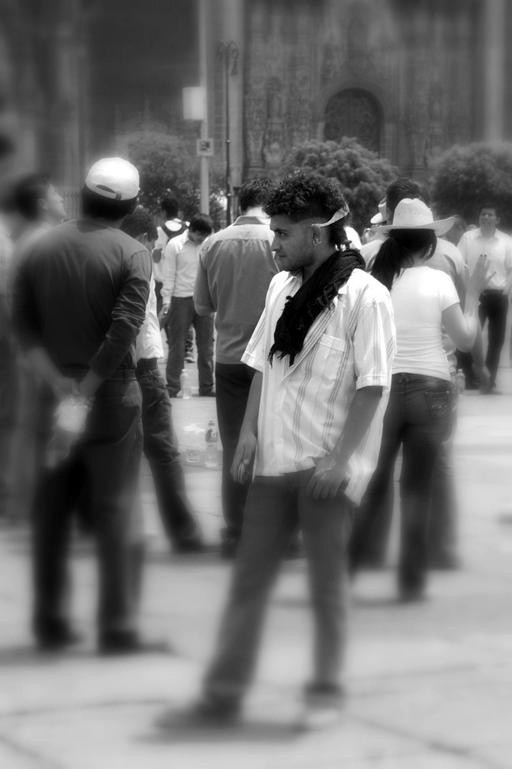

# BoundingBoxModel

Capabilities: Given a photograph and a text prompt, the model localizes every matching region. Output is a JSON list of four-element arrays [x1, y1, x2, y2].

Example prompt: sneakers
[[486, 387, 502, 395], [97, 631, 169, 654], [37, 628, 85, 650], [184, 352, 196, 362]]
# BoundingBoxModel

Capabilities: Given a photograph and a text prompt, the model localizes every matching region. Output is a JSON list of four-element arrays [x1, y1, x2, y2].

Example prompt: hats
[[369, 196, 389, 224], [380, 199, 458, 236], [85, 157, 140, 202]]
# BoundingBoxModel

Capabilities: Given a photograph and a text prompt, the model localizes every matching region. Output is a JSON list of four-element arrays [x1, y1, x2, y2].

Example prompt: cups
[[185, 430, 204, 463]]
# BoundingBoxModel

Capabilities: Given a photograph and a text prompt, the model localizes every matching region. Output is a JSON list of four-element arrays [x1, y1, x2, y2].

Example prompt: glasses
[[311, 201, 350, 227]]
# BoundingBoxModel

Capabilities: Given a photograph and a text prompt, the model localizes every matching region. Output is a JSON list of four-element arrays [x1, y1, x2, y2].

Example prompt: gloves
[[54, 390, 95, 433]]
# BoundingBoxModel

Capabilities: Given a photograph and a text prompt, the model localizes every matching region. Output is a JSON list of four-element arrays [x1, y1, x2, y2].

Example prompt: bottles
[[180, 368, 192, 399], [204, 419, 220, 467], [44, 402, 89, 470], [456, 368, 465, 395]]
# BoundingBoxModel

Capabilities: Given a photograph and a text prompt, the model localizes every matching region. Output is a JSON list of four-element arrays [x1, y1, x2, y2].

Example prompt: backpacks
[[160, 222, 187, 246]]
